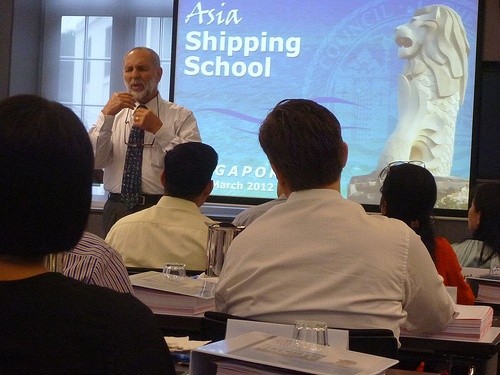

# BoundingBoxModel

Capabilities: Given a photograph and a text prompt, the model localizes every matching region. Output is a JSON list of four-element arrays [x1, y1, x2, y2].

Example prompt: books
[[164, 336, 213, 367], [204, 221, 247, 278], [400, 303, 495, 344], [128, 271, 220, 318], [477, 283, 500, 305], [212, 359, 284, 375]]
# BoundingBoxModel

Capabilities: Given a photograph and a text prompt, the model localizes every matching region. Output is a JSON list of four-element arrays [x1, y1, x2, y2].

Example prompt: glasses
[[388, 160, 425, 177], [124, 120, 156, 148]]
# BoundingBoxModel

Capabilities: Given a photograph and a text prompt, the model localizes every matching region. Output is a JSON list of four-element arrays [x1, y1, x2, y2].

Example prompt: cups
[[292, 319, 329, 352], [163, 263, 187, 281]]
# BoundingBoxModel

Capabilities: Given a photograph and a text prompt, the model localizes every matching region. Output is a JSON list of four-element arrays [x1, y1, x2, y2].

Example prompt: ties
[[120, 104, 150, 212]]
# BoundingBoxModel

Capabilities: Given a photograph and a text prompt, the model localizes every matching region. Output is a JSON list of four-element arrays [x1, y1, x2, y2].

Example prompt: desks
[[124, 265, 500, 375]]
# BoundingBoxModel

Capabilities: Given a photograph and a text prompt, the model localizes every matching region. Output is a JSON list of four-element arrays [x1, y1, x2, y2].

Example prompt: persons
[[45, 228, 136, 298], [231, 174, 289, 230], [86, 46, 203, 230], [212, 98, 460, 349], [105, 140, 220, 270], [449, 178, 500, 269], [378, 159, 476, 306], [0, 93, 176, 375]]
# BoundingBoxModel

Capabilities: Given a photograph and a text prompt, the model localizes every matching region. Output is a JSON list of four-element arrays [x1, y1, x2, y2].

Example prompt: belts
[[109, 193, 163, 203]]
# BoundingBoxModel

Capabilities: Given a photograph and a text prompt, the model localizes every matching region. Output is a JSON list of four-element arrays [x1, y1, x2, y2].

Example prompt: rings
[[135, 116, 139, 122]]
